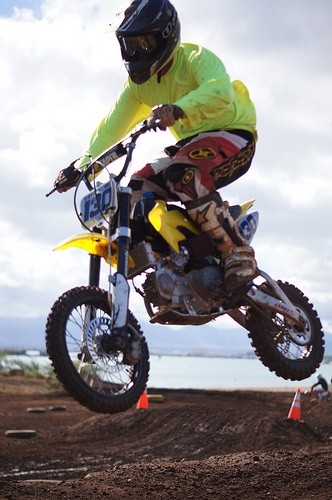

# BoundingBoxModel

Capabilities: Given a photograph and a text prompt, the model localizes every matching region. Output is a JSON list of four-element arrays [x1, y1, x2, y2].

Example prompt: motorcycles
[[47, 114, 325, 414]]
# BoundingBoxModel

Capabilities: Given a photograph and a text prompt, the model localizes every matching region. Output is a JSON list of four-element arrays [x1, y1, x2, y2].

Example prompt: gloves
[[54, 168, 80, 193], [150, 104, 182, 132]]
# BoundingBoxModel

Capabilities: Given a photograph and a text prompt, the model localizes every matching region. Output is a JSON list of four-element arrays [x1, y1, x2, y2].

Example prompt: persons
[[55, 0, 260, 294], [312, 373, 329, 400]]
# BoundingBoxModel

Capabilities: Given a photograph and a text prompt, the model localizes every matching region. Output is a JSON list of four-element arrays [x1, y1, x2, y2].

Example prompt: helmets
[[114, 0, 181, 84]]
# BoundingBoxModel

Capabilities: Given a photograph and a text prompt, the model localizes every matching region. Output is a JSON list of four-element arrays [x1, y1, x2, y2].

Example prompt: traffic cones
[[288, 387, 302, 422], [137, 380, 149, 409]]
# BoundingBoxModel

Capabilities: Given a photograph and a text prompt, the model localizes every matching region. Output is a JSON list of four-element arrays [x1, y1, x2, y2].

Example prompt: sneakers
[[221, 244, 257, 289]]
